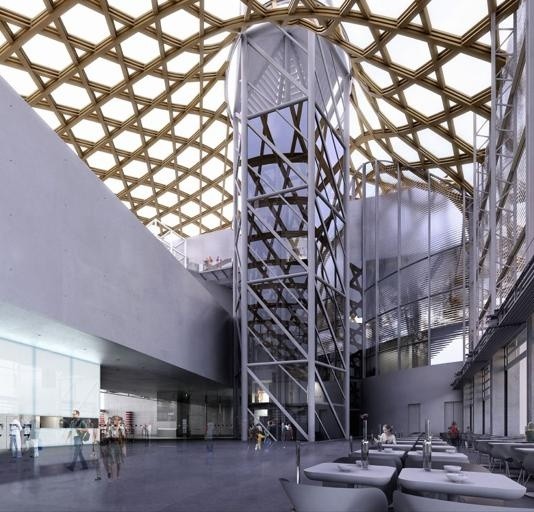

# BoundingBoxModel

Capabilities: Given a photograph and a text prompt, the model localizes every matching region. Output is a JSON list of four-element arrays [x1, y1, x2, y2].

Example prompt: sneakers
[[66, 466, 74, 472], [108, 472, 111, 478], [80, 466, 88, 470], [95, 477, 101, 480]]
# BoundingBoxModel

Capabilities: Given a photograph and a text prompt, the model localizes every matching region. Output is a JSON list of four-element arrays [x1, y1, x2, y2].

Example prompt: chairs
[[277, 431, 534, 512]]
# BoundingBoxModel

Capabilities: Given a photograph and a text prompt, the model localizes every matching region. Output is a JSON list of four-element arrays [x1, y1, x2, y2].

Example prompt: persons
[[448, 421, 459, 446], [216, 256, 222, 269], [204, 418, 216, 453], [247, 423, 294, 451], [0, 418, 35, 459], [377, 424, 397, 445], [64, 410, 128, 481], [207, 256, 213, 268]]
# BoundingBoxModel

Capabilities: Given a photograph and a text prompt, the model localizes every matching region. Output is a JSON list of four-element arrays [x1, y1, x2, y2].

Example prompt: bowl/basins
[[336, 463, 354, 471], [383, 447, 392, 452], [445, 449, 456, 453], [445, 472, 468, 481], [355, 460, 361, 466], [443, 464, 461, 472]]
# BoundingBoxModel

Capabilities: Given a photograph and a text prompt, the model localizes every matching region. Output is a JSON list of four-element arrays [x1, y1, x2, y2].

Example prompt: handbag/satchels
[[75, 419, 90, 441]]
